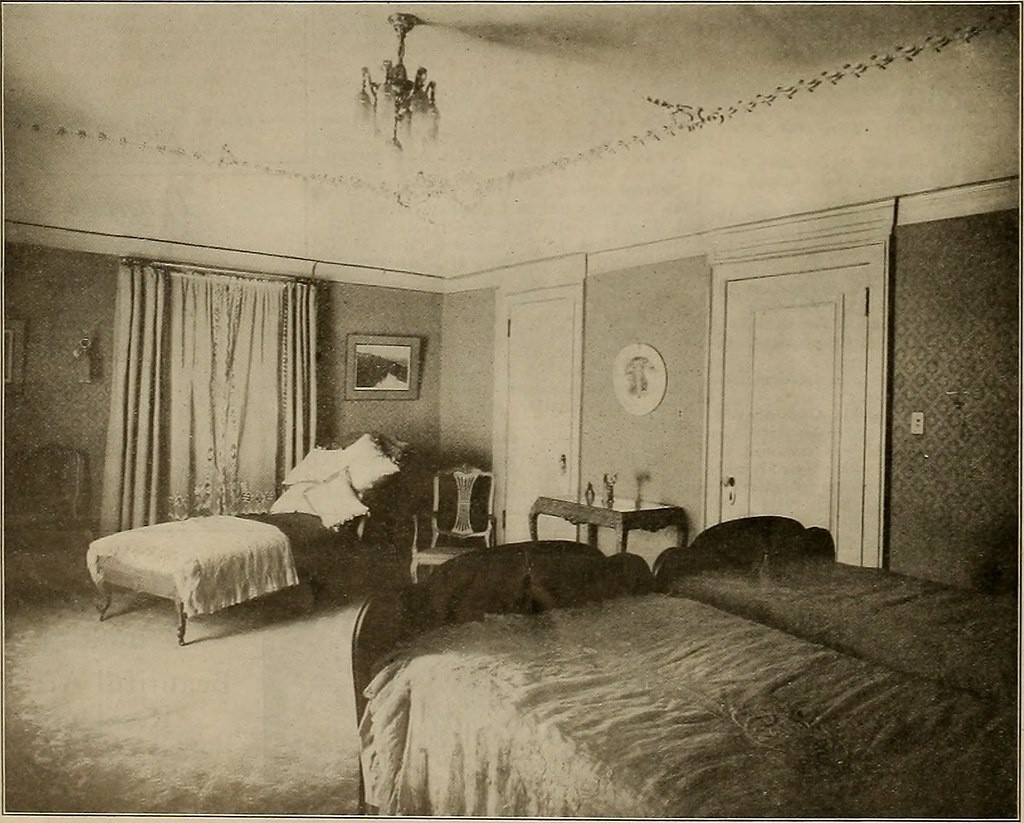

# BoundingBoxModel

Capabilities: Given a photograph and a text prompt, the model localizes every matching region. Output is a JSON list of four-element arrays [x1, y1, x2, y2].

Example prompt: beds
[[351, 542, 1018, 819], [86, 432, 413, 646], [650, 514, 1018, 705]]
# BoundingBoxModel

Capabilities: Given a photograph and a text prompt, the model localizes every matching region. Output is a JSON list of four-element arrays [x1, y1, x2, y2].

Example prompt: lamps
[[944, 377, 971, 410], [356, 61, 441, 155]]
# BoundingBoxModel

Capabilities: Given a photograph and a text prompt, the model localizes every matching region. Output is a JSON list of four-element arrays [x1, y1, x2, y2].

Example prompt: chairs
[[410, 460, 496, 586], [4, 442, 99, 603]]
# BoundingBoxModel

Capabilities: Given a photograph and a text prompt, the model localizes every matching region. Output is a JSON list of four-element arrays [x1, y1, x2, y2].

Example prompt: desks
[[527, 492, 693, 555]]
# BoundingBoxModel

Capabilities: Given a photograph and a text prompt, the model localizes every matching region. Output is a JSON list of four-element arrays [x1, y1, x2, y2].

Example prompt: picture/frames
[[343, 331, 425, 402], [2, 318, 31, 399]]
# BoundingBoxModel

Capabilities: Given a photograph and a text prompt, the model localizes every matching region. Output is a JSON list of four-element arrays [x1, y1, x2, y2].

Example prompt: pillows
[[269, 482, 322, 518], [281, 448, 357, 485], [345, 433, 400, 493], [304, 467, 369, 530]]
